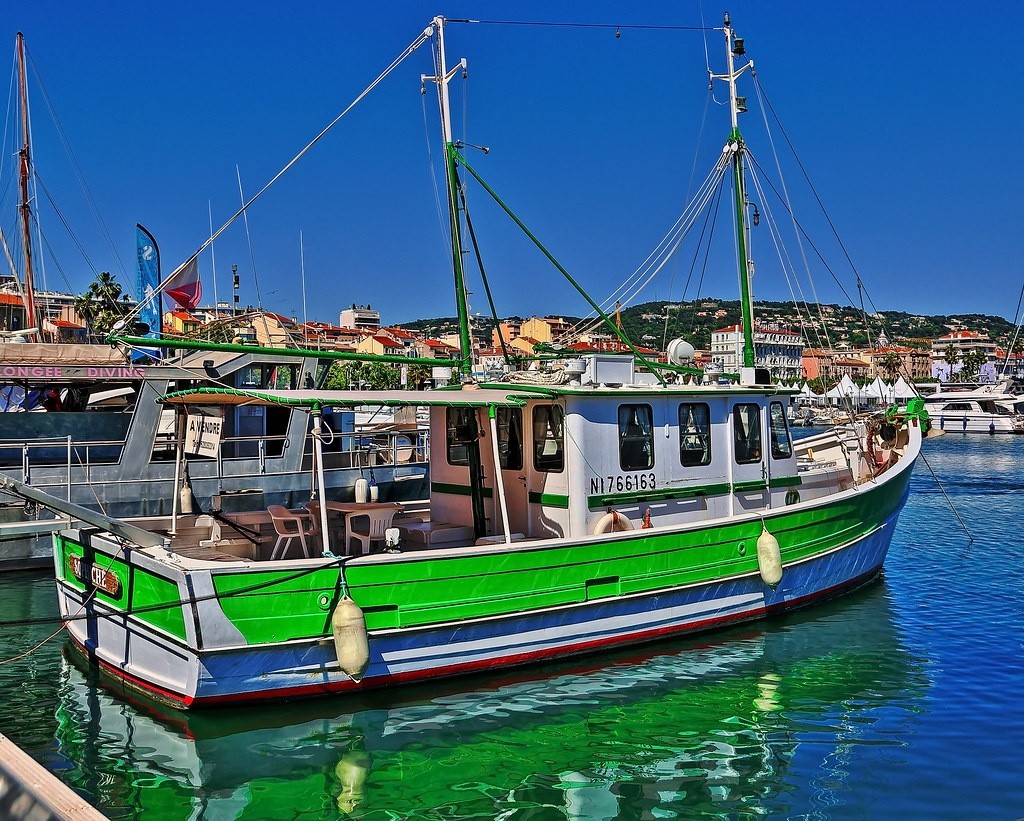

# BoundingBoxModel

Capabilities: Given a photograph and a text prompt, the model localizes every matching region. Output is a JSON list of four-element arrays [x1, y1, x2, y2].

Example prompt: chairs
[[267, 505, 319, 560], [302, 499, 355, 556], [345, 509, 398, 556]]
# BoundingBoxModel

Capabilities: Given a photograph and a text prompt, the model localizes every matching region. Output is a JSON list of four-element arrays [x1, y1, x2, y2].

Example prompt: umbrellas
[[687, 374, 919, 409]]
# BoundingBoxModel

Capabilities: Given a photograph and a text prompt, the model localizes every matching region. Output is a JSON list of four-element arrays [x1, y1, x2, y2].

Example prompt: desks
[[316, 502, 405, 556]]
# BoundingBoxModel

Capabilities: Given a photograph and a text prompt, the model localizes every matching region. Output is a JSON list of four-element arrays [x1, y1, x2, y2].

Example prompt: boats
[[50, 386, 927, 711], [769, 406, 894, 427], [1, 339, 438, 568], [925, 392, 1024, 433]]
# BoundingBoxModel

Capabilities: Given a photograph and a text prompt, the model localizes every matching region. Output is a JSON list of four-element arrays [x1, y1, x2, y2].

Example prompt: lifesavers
[[593, 511, 635, 534]]
